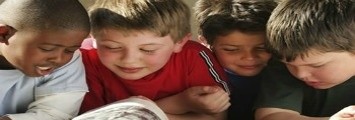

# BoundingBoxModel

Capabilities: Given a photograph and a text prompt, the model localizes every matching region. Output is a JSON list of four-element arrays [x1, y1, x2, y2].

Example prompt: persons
[[0, 0, 91, 120], [192, 0, 278, 120], [255, 0, 355, 120], [78, 0, 230, 120]]
